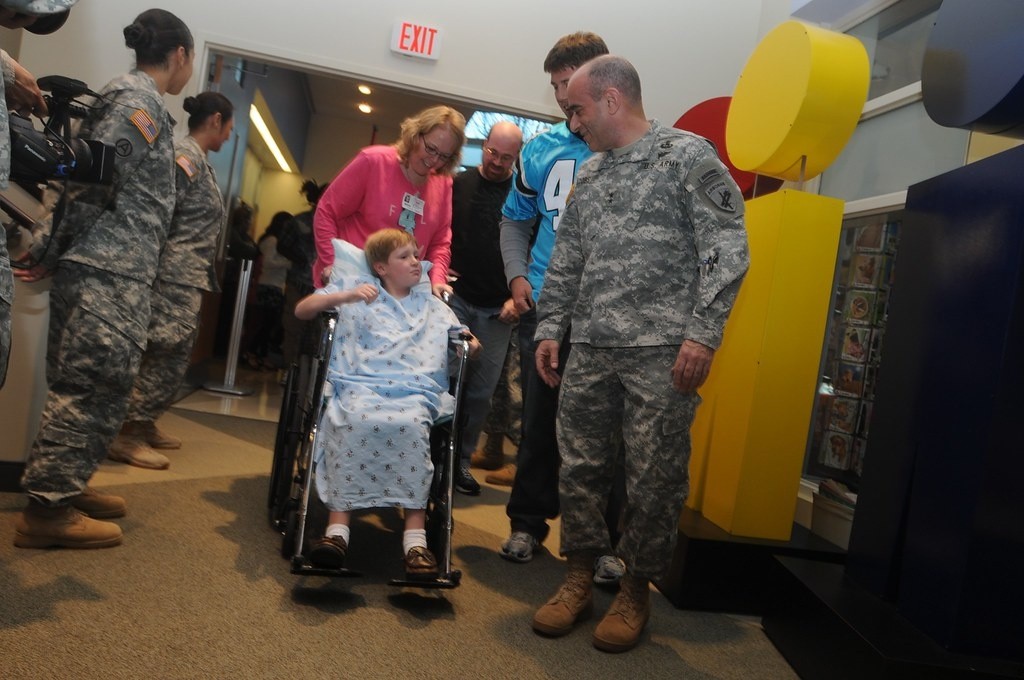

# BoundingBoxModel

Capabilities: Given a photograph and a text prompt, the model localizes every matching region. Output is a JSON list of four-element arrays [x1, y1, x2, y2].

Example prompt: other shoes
[[470, 449, 505, 470], [485, 463, 518, 486]]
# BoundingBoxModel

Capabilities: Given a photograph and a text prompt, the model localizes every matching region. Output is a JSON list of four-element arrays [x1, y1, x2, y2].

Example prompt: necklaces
[[405, 165, 428, 196]]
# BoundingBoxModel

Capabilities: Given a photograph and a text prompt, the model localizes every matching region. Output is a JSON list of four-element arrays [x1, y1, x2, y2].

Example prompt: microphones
[[36, 75, 102, 97]]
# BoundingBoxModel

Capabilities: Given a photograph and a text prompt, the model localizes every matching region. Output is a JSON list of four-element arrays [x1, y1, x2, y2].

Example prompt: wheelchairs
[[267, 290, 473, 590]]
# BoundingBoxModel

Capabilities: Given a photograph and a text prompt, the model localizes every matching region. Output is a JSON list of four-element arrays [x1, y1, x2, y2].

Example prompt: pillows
[[328, 239, 435, 309]]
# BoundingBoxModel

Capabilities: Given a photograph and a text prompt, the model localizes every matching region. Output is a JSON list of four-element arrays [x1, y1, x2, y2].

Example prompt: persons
[[499, 31, 631, 583], [314, 106, 466, 300], [14, 8, 196, 549], [294, 226, 482, 579], [214, 176, 329, 385], [535, 55, 751, 655], [108, 90, 235, 472], [0, 50, 48, 398], [450, 120, 541, 496]]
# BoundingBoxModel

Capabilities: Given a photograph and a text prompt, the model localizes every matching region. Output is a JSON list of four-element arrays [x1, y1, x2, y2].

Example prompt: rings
[[510, 319, 513, 322]]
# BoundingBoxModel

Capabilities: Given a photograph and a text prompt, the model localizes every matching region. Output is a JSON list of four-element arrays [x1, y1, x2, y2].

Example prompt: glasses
[[422, 134, 453, 163]]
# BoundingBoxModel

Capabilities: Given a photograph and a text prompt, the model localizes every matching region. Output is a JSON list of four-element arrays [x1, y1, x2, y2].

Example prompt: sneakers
[[14, 504, 121, 549], [593, 588, 650, 653], [70, 488, 126, 517], [593, 555, 624, 584], [108, 435, 170, 470], [499, 532, 542, 562], [533, 572, 592, 636], [402, 546, 439, 580], [308, 535, 347, 567], [454, 465, 481, 494], [121, 423, 182, 449]]
